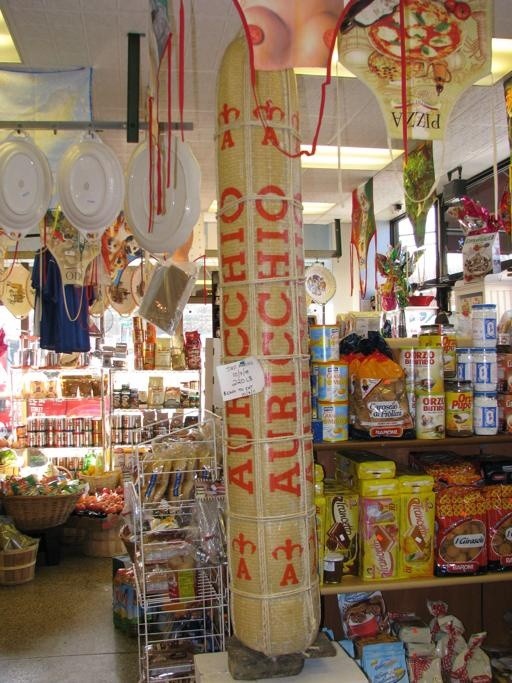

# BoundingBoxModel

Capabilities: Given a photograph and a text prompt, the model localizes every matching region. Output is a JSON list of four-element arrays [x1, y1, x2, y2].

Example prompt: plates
[[125, 131, 203, 254], [107, 264, 140, 317], [1, 129, 55, 241], [57, 130, 124, 244], [132, 260, 155, 305], [305, 261, 337, 306]]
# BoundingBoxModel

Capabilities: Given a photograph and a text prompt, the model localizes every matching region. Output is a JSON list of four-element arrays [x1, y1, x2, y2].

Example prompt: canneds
[[309, 325, 350, 442], [16, 414, 146, 448], [400, 304, 512, 437], [133, 316, 154, 370]]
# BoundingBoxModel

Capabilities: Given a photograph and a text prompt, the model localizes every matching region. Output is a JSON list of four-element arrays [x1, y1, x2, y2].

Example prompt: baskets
[[1, 464, 85, 533], [77, 466, 124, 495]]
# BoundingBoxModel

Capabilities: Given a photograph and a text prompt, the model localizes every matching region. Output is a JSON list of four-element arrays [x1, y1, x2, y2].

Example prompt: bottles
[[111, 568, 159, 637]]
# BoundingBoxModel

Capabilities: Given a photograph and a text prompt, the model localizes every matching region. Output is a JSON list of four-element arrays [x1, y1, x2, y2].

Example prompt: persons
[[237, 0, 345, 72]]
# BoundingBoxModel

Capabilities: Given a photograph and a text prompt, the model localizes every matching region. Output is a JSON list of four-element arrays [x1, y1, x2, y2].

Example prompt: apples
[[76, 486, 125, 513]]
